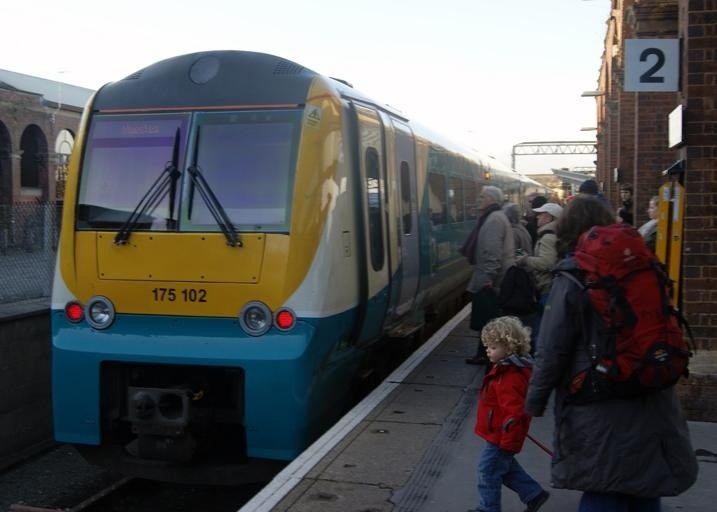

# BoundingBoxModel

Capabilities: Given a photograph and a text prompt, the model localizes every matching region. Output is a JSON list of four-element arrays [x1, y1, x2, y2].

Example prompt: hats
[[532, 203, 564, 218]]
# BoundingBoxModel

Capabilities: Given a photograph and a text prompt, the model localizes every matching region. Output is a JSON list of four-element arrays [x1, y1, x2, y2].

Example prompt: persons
[[636, 194, 661, 254], [567, 190, 577, 201], [467, 316, 551, 512], [460, 186, 515, 364], [617, 184, 635, 227], [552, 191, 559, 203], [503, 202, 532, 315], [522, 196, 699, 512], [522, 195, 548, 247], [515, 203, 565, 358], [579, 179, 610, 209]]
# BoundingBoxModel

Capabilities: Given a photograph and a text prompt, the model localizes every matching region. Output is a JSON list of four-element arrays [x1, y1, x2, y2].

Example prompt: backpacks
[[557, 220, 698, 405]]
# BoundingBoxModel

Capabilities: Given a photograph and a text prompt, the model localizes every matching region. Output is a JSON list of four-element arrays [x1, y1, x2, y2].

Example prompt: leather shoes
[[465, 354, 480, 364]]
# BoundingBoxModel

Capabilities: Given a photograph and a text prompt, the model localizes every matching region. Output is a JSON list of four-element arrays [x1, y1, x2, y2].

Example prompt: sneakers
[[524, 489, 548, 512]]
